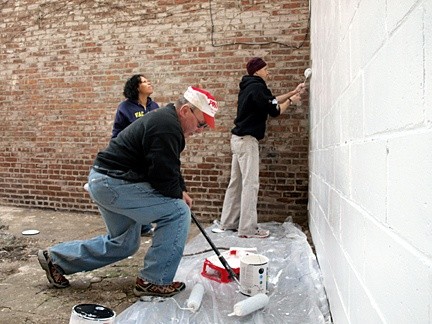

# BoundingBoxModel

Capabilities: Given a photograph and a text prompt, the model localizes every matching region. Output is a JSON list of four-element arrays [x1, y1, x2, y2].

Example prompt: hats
[[246, 57, 267, 76], [184, 85, 218, 129]]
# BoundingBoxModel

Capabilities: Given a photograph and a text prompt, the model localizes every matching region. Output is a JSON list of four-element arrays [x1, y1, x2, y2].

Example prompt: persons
[[219, 58, 305, 238], [112, 75, 160, 237], [37, 86, 219, 297]]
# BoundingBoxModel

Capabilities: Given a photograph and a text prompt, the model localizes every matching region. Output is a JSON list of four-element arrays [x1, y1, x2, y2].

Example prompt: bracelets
[[288, 98, 294, 106]]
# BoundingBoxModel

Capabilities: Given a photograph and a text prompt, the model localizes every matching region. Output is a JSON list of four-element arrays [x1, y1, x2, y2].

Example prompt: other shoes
[[132, 277, 186, 298], [141, 227, 158, 237], [37, 249, 70, 289]]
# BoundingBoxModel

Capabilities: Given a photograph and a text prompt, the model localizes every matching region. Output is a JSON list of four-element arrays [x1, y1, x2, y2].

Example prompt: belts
[[93, 166, 130, 181]]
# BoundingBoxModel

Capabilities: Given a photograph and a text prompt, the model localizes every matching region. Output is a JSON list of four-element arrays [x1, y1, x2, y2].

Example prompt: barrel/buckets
[[239, 252, 268, 297], [69, 302, 116, 324]]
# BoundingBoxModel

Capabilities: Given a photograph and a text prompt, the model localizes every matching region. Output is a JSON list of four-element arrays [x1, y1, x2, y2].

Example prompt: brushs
[[140, 283, 204, 312], [191, 213, 270, 316], [304, 67, 312, 84]]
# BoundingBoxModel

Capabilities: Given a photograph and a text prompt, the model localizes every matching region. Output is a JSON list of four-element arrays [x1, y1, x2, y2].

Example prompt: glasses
[[190, 108, 213, 129]]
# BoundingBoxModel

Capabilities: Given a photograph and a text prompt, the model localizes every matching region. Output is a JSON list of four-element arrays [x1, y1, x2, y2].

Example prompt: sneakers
[[238, 227, 271, 239]]
